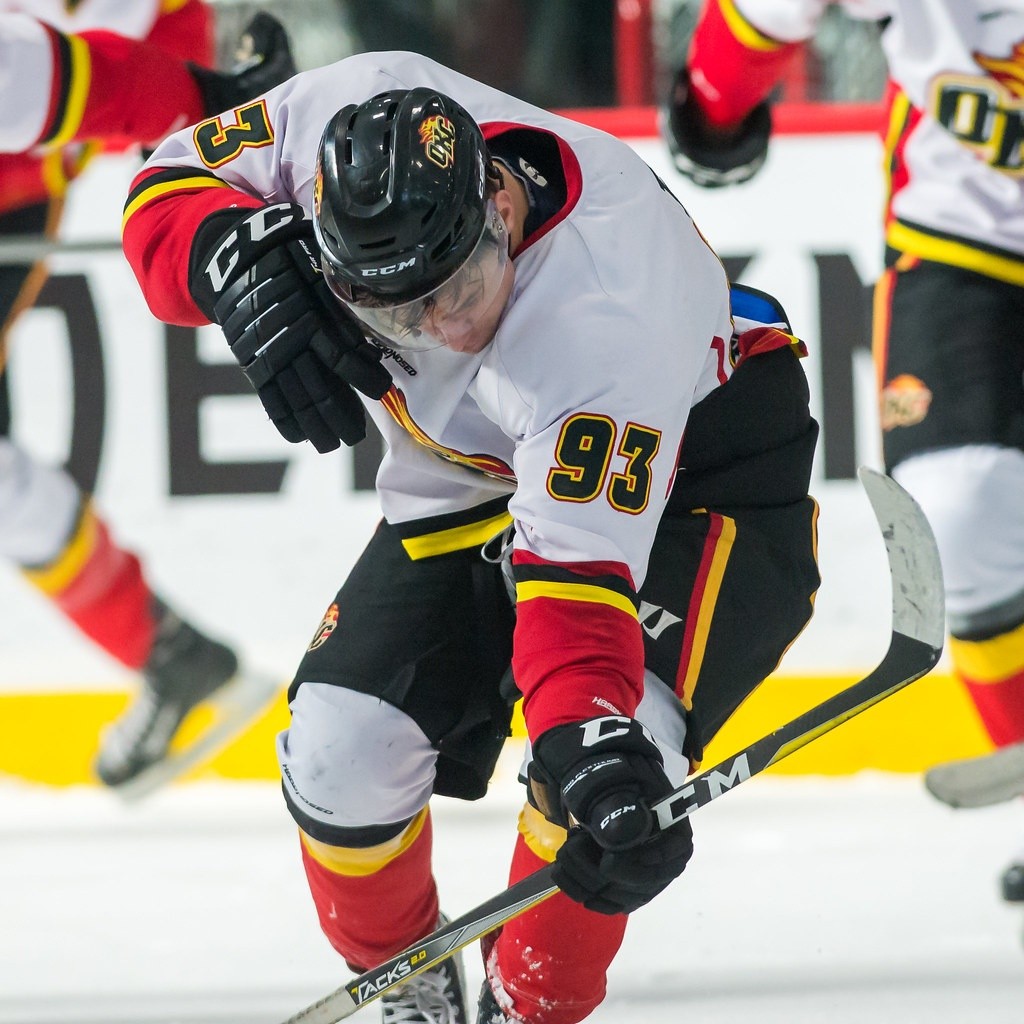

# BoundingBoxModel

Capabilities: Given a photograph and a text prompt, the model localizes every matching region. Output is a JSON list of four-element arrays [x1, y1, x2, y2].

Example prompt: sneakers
[[379, 910, 468, 1024], [94, 594, 286, 801], [478, 933, 521, 1024]]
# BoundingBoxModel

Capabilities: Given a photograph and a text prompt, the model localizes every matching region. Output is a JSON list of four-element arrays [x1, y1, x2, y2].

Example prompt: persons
[[659, 0, 1024, 810], [123, 50, 821, 1024], [0, 0, 293, 791]]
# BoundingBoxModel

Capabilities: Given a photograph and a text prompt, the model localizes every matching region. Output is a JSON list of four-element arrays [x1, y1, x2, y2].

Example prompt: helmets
[[310, 89, 492, 311]]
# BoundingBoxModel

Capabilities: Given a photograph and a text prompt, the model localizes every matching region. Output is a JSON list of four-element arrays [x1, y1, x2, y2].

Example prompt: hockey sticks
[[275, 466, 948, 1023]]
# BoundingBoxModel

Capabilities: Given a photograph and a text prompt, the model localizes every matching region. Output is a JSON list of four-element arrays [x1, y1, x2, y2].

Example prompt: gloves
[[518, 712, 694, 917], [194, 205, 389, 454], [659, 57, 783, 187]]
